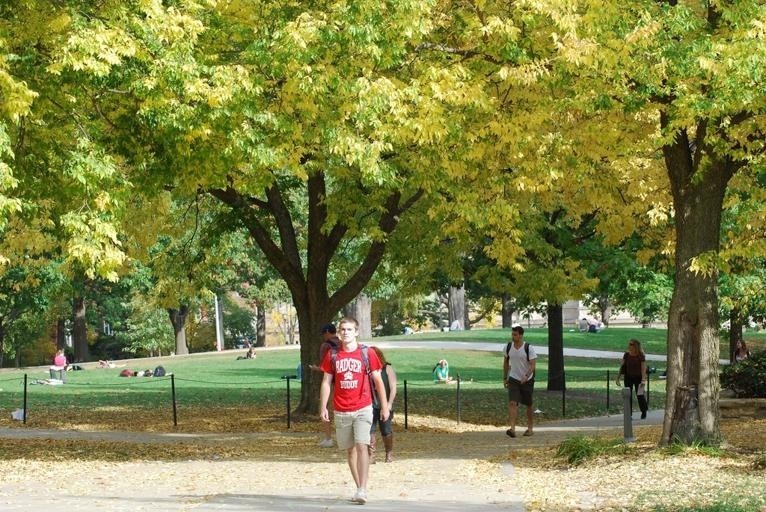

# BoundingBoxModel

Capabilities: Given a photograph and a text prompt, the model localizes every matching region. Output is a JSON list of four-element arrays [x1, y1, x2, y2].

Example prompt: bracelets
[[504, 377, 507, 380], [641, 379, 645, 381], [525, 373, 530, 378]]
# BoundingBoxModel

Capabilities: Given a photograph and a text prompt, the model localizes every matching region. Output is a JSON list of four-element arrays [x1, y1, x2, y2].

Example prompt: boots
[[382, 433, 393, 463], [368, 434, 376, 464]]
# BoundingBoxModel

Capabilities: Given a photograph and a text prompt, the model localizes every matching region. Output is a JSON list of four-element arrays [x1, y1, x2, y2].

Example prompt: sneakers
[[506, 429, 515, 437], [523, 429, 534, 436]]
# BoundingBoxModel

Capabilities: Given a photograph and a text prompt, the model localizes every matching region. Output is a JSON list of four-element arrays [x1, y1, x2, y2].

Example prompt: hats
[[319, 324, 335, 335]]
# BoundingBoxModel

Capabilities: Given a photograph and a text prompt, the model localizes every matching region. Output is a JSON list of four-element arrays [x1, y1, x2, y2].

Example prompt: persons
[[580, 317, 606, 333], [247, 348, 256, 359], [733, 339, 752, 364], [368, 347, 397, 465], [434, 360, 453, 384], [503, 326, 537, 437], [616, 339, 648, 419], [320, 317, 390, 504], [309, 324, 342, 447], [50, 349, 172, 381]]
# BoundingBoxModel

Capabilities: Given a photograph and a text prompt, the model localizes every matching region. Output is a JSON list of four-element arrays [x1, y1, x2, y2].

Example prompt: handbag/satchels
[[119, 366, 166, 377]]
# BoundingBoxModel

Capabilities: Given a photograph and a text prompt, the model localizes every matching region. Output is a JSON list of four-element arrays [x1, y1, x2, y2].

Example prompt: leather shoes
[[640, 411, 646, 419]]
